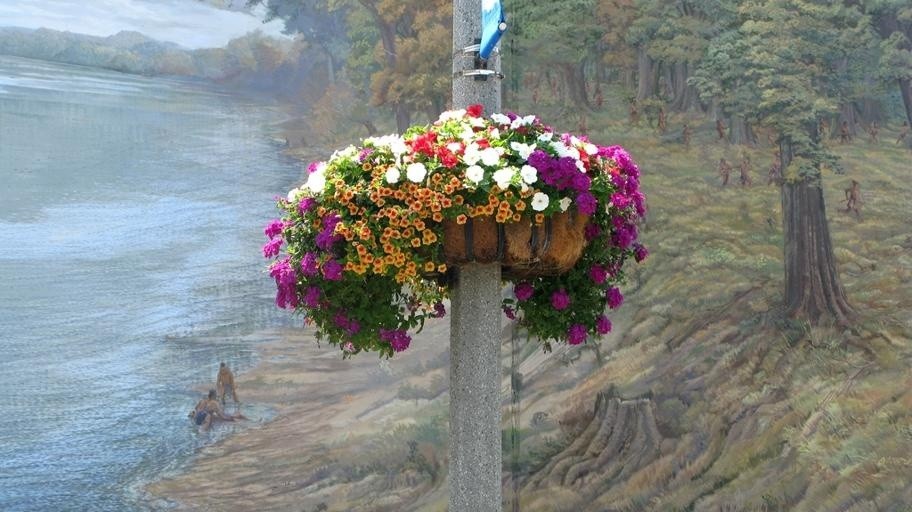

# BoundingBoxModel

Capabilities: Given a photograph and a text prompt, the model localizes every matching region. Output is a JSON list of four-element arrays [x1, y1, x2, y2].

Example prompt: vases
[[418, 206, 597, 280]]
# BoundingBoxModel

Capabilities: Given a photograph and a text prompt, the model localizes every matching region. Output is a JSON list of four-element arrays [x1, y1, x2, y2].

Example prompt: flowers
[[259, 103, 648, 363]]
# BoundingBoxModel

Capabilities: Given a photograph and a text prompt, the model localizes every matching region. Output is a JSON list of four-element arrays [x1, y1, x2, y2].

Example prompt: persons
[[515, 70, 910, 217], [194, 363, 239, 434]]
